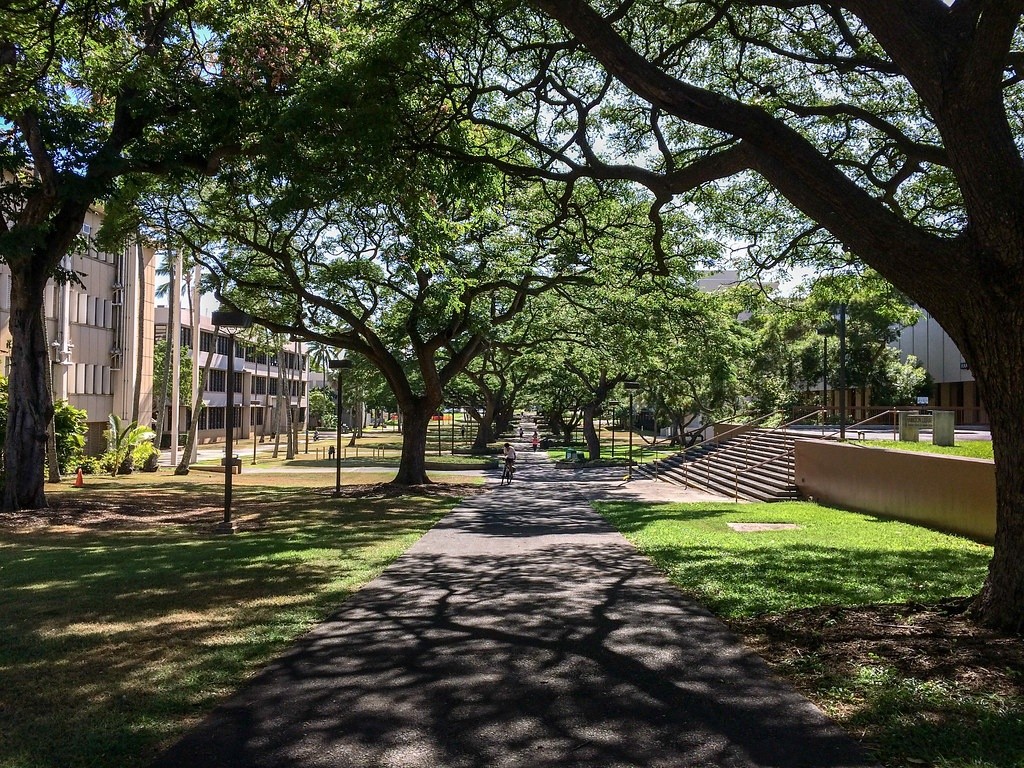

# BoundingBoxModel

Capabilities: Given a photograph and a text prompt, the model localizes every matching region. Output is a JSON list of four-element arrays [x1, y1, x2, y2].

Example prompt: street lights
[[328, 361, 354, 491], [609, 401, 620, 457], [211, 311, 253, 521], [623, 382, 640, 481], [450, 395, 457, 455]]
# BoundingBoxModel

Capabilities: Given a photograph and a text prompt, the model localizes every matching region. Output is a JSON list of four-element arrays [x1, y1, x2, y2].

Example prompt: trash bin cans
[[665, 427, 674, 438], [221, 454, 242, 474]]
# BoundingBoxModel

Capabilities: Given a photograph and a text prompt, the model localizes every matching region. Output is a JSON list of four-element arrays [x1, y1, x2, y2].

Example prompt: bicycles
[[500, 460, 515, 486], [313, 434, 319, 441]]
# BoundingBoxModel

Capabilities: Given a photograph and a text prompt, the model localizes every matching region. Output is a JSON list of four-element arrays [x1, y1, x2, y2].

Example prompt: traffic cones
[[71, 470, 86, 488]]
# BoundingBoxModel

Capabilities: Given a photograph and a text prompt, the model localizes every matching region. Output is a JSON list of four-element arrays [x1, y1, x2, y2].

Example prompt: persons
[[531, 436, 539, 452], [520, 428, 523, 438], [504, 443, 516, 480], [314, 430, 319, 436], [534, 431, 537, 438], [460, 425, 465, 437]]
